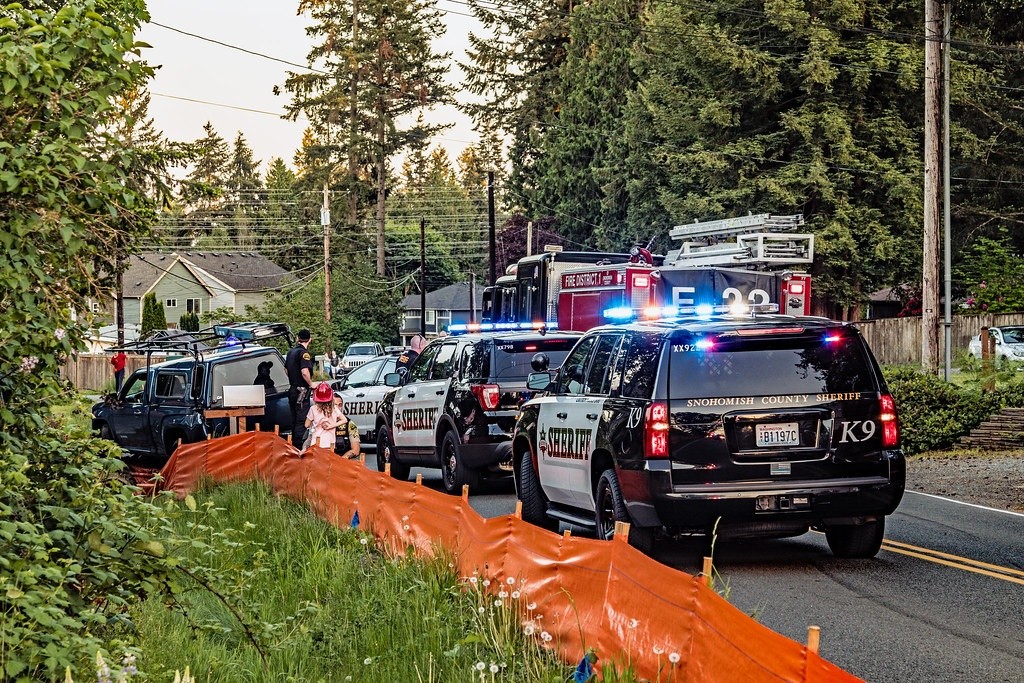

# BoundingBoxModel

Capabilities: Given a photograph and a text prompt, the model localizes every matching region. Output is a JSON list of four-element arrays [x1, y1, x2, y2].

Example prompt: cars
[[301, 346, 422, 444], [967, 325, 1024, 373]]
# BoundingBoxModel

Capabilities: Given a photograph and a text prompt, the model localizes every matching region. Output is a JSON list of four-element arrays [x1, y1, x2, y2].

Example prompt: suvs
[[514, 302, 908, 558], [90, 322, 302, 463], [375, 321, 587, 493], [340, 342, 385, 371]]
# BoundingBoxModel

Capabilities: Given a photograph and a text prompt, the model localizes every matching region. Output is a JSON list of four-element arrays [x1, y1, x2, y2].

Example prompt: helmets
[[313, 382, 333, 402]]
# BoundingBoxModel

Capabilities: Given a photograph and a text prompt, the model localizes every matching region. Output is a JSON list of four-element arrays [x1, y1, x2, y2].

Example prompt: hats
[[299, 329, 310, 339], [257, 360, 273, 371]]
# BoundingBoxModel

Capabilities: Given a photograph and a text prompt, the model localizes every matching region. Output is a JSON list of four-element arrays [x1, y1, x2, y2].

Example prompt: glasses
[[414, 333, 423, 346]]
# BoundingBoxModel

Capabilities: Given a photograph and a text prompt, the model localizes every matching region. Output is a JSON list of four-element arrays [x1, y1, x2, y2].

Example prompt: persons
[[332, 392, 362, 463], [396, 334, 427, 378], [323, 352, 331, 376], [305, 381, 348, 451], [330, 351, 341, 379], [110, 347, 130, 393], [283, 329, 316, 450]]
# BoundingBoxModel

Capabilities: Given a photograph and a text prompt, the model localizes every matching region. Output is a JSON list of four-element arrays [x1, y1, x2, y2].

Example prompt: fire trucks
[[481, 213, 814, 331]]
